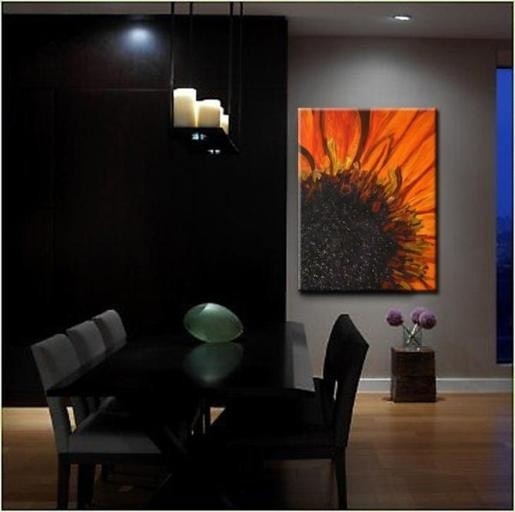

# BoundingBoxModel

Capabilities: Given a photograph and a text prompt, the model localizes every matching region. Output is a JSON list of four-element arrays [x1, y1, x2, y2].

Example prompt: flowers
[[385, 306, 437, 329]]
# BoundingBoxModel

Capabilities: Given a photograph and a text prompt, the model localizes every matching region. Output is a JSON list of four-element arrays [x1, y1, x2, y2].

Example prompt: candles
[[173, 87, 230, 138]]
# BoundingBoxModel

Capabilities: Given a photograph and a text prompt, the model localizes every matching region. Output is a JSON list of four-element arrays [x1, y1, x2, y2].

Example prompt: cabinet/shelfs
[[391, 347, 436, 402]]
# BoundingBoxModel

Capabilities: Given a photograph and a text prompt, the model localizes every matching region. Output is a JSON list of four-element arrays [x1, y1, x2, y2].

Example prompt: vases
[[403, 325, 422, 350]]
